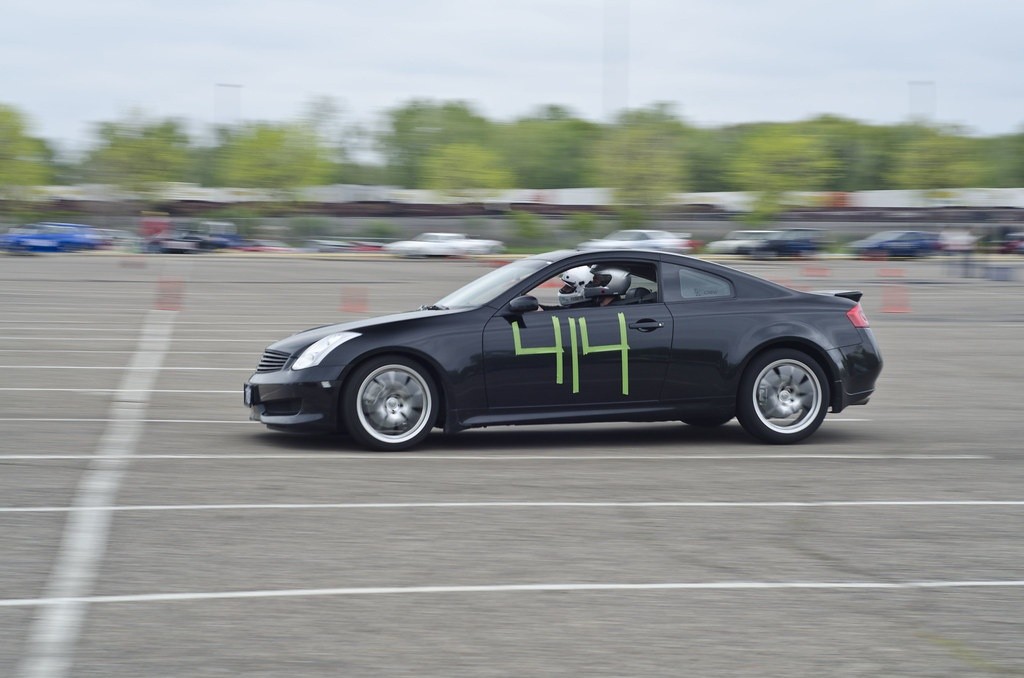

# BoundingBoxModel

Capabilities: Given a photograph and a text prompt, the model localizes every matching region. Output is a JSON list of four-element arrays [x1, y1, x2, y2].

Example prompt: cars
[[382, 234, 501, 256], [0, 222, 110, 254], [578, 230, 693, 255], [243, 247, 883, 453], [848, 231, 945, 259], [706, 230, 776, 255], [736, 229, 829, 258]]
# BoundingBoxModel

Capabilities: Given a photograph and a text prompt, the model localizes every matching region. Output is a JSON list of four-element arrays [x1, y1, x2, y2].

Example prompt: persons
[[559, 265, 596, 308], [585, 264, 632, 306]]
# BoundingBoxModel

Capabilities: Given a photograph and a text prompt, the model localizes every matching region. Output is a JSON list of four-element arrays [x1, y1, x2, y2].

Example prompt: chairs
[[624, 287, 652, 304]]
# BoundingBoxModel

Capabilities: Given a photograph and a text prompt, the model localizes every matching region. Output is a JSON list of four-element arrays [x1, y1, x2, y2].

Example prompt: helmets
[[584, 264, 632, 300], [557, 265, 594, 307]]
